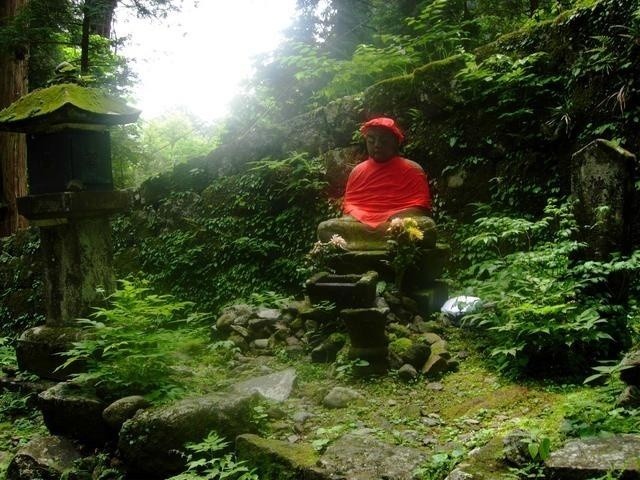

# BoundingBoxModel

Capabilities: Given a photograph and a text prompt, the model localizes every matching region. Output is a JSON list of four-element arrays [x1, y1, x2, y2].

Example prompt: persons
[[313, 115, 440, 255]]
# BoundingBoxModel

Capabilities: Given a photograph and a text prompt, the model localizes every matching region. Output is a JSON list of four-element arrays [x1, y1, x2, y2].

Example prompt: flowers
[[380, 217, 424, 290], [301, 233, 347, 273]]
